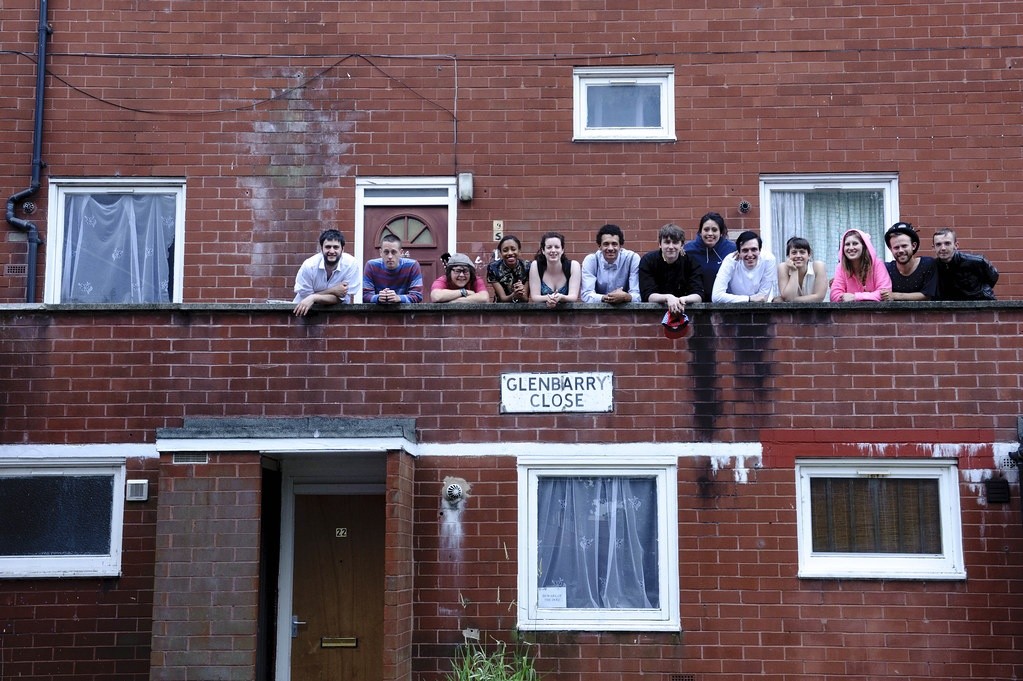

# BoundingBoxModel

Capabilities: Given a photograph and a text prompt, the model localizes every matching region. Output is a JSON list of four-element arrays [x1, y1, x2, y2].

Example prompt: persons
[[363, 234, 423, 303], [931, 227, 1000, 302], [828, 228, 892, 300], [638, 223, 705, 313], [429, 253, 489, 303], [528, 232, 581, 309], [829, 221, 940, 301], [581, 223, 642, 304], [678, 211, 742, 289], [776, 237, 827, 303], [485, 235, 531, 303], [711, 230, 777, 302], [292, 230, 361, 316]]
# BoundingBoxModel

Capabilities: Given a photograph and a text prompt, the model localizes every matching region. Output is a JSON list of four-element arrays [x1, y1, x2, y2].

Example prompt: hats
[[440, 252, 476, 270], [884, 222, 920, 255], [661, 311, 690, 339]]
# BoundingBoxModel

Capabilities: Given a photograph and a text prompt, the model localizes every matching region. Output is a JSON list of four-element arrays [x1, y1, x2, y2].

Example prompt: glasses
[[451, 268, 470, 275]]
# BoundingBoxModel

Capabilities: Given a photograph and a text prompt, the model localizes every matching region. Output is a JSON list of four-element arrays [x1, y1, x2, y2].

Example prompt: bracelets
[[511, 294, 518, 303], [749, 295, 751, 302]]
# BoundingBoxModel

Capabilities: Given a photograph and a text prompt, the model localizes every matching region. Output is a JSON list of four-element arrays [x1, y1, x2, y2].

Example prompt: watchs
[[460, 288, 466, 296]]
